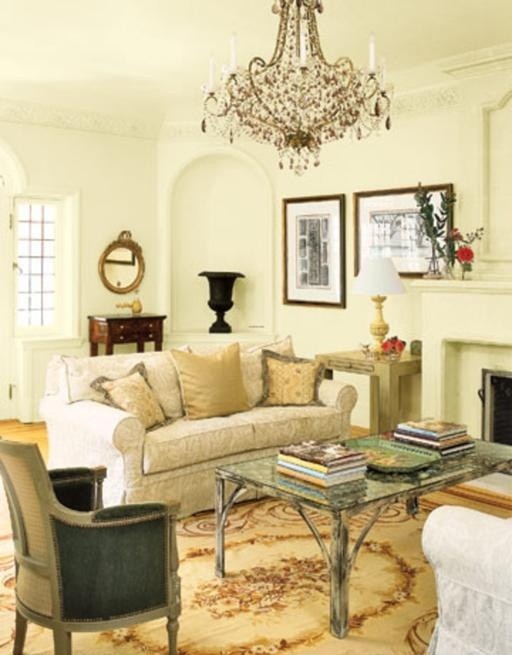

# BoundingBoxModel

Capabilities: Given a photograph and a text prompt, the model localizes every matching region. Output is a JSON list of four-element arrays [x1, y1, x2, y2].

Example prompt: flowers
[[411, 178, 486, 260], [455, 247, 474, 280]]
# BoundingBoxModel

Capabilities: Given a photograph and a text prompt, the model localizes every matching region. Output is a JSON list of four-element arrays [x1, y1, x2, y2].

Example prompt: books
[[391, 417, 477, 461], [278, 437, 369, 492]]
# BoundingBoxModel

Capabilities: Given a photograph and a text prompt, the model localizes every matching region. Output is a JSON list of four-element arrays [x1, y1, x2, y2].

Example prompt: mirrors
[[97, 229, 146, 294]]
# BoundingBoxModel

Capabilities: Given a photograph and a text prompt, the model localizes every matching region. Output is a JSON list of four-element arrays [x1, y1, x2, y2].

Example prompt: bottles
[[131, 290, 143, 313]]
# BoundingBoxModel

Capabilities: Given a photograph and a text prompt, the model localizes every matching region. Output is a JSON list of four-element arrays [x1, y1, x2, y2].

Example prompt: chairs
[[419, 504, 512, 655], [0, 436, 182, 654]]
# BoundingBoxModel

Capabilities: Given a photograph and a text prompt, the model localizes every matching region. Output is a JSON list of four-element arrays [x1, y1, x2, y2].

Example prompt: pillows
[[89, 362, 174, 432], [169, 341, 250, 422], [131, 344, 195, 421], [240, 335, 297, 410], [260, 349, 327, 407]]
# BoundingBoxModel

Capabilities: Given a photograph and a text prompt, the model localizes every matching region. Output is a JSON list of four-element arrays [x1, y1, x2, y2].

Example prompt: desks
[[87, 312, 167, 357], [315, 348, 421, 437], [212, 426, 511, 639]]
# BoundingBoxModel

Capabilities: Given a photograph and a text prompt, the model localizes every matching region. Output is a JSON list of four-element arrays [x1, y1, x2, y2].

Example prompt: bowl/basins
[[362, 350, 403, 360]]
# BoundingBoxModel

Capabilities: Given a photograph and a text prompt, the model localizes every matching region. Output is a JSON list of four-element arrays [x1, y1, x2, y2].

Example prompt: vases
[[197, 270, 246, 334], [422, 246, 443, 279]]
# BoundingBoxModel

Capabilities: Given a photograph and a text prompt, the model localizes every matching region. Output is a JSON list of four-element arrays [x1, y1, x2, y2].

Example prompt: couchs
[[37, 335, 358, 521]]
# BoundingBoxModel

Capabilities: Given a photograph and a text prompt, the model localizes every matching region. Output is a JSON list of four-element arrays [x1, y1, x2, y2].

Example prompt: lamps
[[349, 255, 407, 356], [198, 0, 393, 176]]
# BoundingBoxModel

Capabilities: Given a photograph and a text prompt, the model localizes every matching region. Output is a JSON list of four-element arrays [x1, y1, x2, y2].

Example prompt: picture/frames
[[352, 182, 455, 280], [281, 192, 347, 310]]
